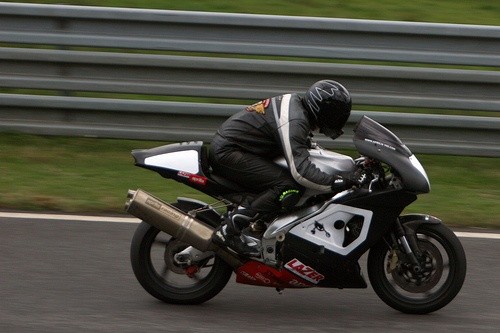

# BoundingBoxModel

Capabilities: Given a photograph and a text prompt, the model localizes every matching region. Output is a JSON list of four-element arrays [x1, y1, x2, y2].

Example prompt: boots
[[210, 198, 262, 258]]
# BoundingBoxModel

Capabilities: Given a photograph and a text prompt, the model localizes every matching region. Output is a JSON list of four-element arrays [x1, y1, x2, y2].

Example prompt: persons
[[209, 80, 367, 257]]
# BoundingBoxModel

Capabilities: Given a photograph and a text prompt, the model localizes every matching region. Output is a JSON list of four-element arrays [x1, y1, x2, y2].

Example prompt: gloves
[[332, 166, 373, 191]]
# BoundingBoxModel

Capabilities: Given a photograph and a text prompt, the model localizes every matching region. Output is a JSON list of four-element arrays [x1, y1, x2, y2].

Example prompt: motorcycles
[[123, 114, 467, 315]]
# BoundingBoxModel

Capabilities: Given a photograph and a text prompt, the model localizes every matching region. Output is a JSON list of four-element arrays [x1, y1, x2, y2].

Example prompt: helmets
[[302, 79, 353, 141]]
